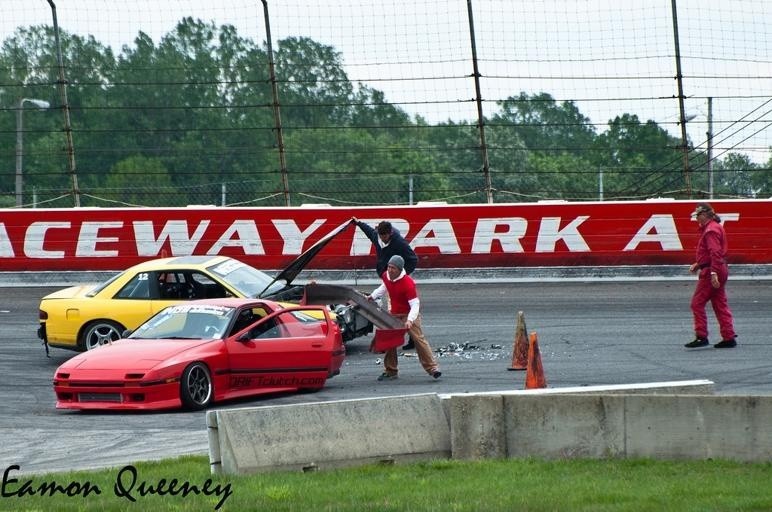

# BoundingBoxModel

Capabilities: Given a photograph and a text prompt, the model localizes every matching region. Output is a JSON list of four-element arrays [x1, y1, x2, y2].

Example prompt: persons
[[351, 214, 421, 352], [684, 201, 739, 350], [364, 254, 442, 381]]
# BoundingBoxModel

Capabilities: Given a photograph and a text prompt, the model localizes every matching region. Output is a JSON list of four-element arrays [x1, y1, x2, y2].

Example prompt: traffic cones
[[504, 308, 529, 374], [521, 329, 550, 389]]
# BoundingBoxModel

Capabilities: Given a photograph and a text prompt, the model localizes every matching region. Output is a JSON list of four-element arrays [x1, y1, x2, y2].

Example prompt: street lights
[[14, 95, 52, 207]]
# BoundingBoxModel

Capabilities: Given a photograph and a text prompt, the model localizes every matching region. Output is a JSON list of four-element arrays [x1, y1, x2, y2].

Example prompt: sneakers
[[402, 343, 415, 351], [714, 339, 738, 348], [376, 372, 399, 382], [684, 336, 710, 349], [433, 369, 442, 379]]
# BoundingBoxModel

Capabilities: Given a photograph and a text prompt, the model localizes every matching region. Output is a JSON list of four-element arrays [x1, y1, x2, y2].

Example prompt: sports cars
[[48, 293, 349, 415], [34, 217, 378, 354]]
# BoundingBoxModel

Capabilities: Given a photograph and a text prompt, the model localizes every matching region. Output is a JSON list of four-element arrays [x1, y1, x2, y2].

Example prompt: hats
[[690, 202, 712, 218], [386, 254, 405, 271]]
